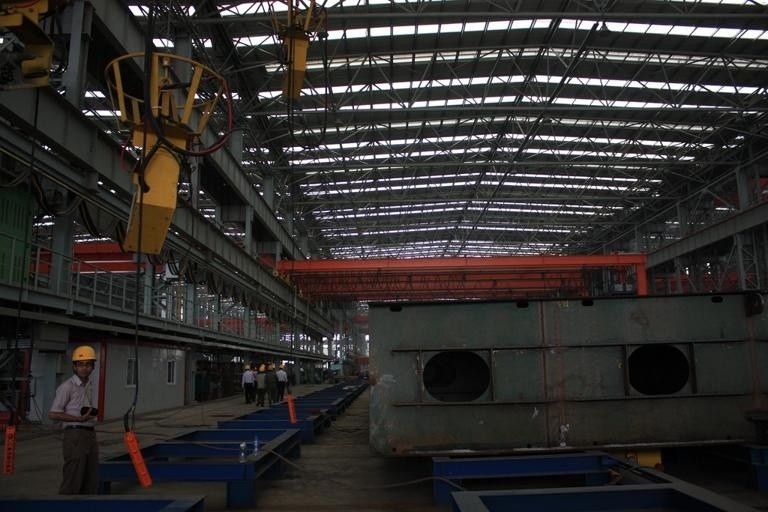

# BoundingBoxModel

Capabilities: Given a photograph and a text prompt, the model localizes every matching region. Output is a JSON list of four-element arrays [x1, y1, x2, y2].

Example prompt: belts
[[66, 425, 94, 430]]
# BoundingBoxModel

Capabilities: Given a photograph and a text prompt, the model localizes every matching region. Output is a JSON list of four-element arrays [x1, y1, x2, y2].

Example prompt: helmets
[[245, 363, 285, 372], [72, 346, 97, 362]]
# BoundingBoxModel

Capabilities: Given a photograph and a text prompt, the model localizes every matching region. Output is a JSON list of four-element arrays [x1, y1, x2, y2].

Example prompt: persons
[[240, 359, 287, 407], [47, 346, 104, 496]]
